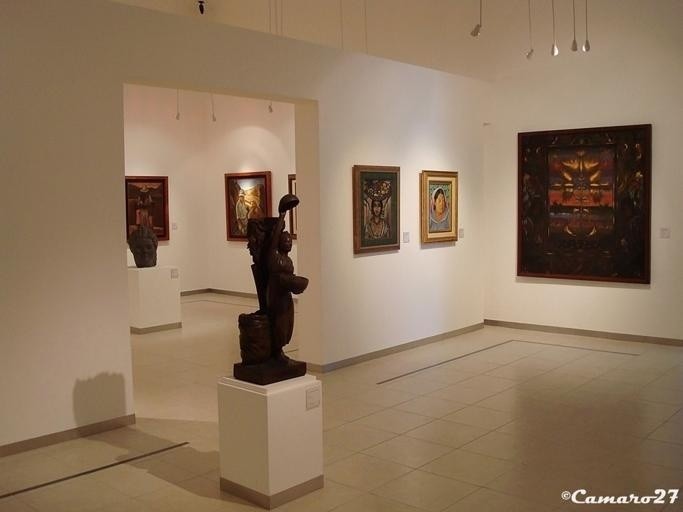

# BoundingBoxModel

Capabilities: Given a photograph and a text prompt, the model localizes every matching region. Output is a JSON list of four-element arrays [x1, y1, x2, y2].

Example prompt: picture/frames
[[354, 165, 400, 254], [125, 176, 169, 240], [517, 124, 652, 284], [288, 174, 297, 238], [224, 171, 272, 241], [422, 170, 458, 242]]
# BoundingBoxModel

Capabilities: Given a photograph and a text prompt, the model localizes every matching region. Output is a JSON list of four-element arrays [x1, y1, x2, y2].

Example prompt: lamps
[[552, 0, 559, 56], [471, 0, 483, 37], [526, 0, 534, 59], [572, 0, 578, 51], [583, 0, 590, 52]]
